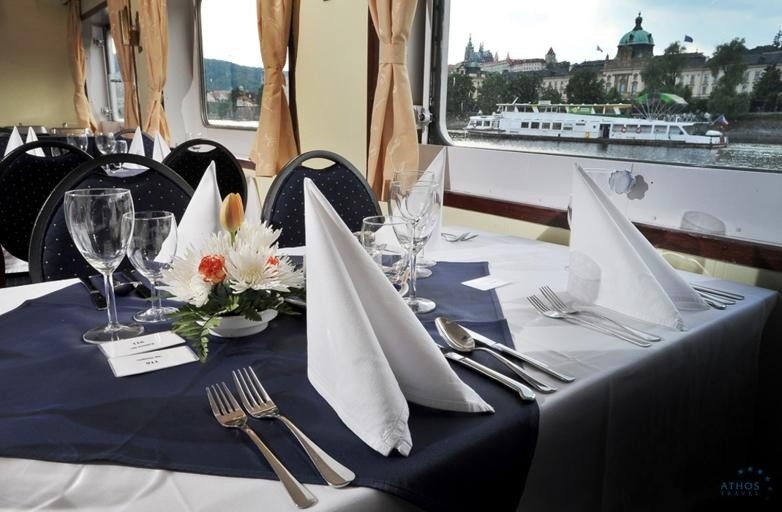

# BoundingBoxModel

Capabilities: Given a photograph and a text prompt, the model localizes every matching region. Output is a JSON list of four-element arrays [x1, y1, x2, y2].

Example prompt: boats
[[466, 92, 734, 149]]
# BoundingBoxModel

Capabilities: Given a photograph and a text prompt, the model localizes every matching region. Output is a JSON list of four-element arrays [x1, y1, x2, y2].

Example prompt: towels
[[374, 148, 446, 251], [566, 162, 709, 331], [303, 177, 495, 457]]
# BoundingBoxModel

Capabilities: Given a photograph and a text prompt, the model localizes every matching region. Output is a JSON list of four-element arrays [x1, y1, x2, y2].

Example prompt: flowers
[[144, 194, 303, 362]]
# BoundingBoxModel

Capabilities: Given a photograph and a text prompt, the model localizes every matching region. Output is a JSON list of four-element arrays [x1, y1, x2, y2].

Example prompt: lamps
[[119, 9, 141, 52]]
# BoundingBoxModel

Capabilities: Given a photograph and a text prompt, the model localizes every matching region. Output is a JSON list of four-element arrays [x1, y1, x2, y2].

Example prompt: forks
[[527, 283, 662, 348], [205, 382, 320, 509], [229, 364, 359, 490]]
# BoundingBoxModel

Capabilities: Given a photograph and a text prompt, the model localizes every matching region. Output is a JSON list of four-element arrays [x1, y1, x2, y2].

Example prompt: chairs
[[0, 124, 249, 284], [262, 150, 385, 250]]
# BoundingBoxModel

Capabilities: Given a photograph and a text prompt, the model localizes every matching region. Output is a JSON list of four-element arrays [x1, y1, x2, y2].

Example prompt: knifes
[[71, 258, 152, 313]]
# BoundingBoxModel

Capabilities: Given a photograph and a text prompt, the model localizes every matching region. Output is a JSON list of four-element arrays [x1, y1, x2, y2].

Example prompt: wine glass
[[353, 170, 437, 316], [121, 210, 181, 324], [563, 167, 632, 277], [67, 132, 129, 174], [63, 186, 145, 345]]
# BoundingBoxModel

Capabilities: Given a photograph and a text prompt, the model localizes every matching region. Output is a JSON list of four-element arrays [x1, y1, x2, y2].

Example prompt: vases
[[195, 308, 277, 337]]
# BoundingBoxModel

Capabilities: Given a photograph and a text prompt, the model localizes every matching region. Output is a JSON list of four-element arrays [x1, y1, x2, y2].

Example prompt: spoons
[[421, 315, 577, 403]]
